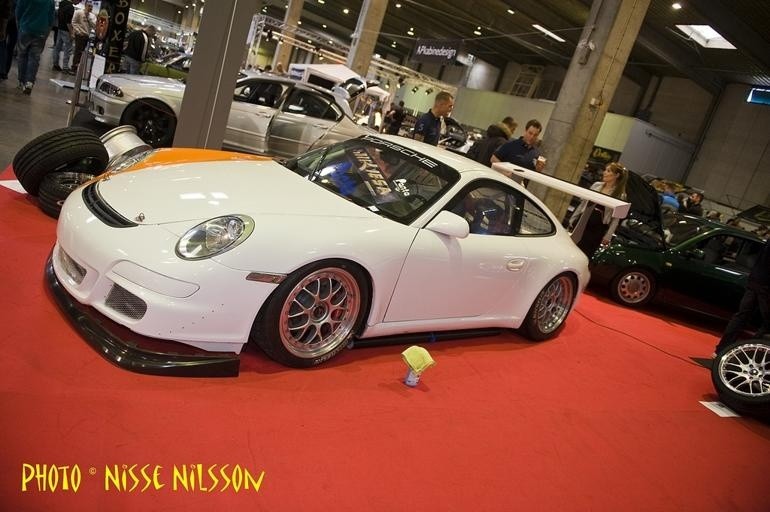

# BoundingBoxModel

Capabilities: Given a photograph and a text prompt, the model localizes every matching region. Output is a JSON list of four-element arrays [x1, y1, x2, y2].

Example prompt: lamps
[[313, 45, 325, 61], [384, 78, 434, 96]]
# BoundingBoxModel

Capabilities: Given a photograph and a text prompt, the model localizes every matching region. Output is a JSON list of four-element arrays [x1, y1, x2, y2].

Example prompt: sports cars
[[44, 133, 632, 377]]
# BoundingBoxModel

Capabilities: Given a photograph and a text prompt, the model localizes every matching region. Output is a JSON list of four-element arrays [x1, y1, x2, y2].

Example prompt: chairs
[[453, 195, 508, 233], [700, 239, 760, 269]]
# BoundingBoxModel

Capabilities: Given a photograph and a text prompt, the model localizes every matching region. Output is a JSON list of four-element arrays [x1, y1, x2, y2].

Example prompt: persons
[[650, 177, 721, 222], [466, 116, 518, 167], [568, 162, 629, 258], [490, 118, 548, 183], [121, 26, 156, 75], [276, 62, 289, 78], [262, 66, 273, 74], [378, 102, 394, 133], [0, 2, 96, 95], [384, 100, 405, 135], [252, 65, 261, 75], [412, 91, 454, 147]]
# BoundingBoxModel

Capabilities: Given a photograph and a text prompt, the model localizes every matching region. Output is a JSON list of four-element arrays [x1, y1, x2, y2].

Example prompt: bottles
[[403, 364, 422, 387], [536, 156, 546, 172]]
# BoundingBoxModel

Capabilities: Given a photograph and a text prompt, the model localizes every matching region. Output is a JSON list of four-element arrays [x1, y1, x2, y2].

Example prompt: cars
[[589, 214, 769, 333], [409, 115, 474, 155], [89, 36, 194, 83], [88, 70, 378, 158]]
